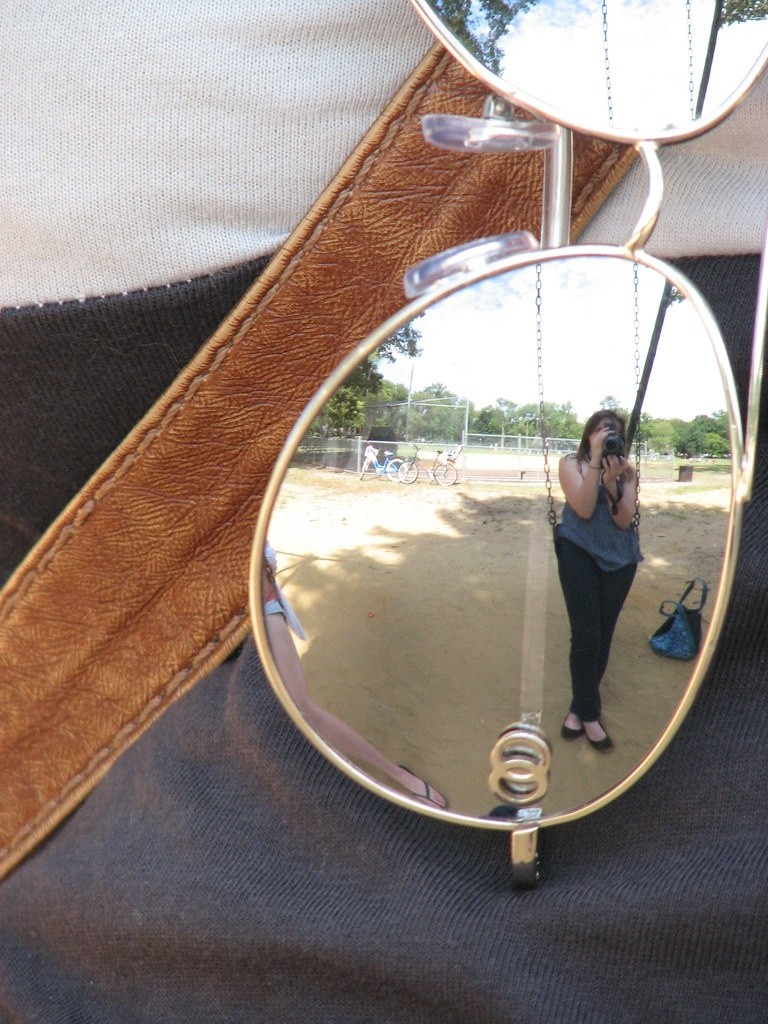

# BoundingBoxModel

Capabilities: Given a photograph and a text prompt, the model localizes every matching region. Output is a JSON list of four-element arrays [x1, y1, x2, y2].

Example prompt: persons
[[555, 411, 644, 752], [265, 540, 448, 811]]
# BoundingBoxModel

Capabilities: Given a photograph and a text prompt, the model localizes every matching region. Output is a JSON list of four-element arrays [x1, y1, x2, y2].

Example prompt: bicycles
[[398, 443, 463, 487], [360, 443, 409, 484]]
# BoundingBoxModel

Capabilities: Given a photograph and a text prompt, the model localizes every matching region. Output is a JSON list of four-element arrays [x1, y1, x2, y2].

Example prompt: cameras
[[603, 436, 624, 468]]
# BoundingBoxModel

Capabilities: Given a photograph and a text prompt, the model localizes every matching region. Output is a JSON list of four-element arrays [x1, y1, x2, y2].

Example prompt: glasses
[[252, 0, 768, 884]]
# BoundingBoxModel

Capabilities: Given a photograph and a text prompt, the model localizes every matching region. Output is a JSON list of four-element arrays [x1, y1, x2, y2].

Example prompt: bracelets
[[589, 465, 601, 470]]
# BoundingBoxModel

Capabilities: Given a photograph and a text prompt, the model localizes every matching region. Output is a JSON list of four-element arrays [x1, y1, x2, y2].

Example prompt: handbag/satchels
[[649, 577, 707, 660]]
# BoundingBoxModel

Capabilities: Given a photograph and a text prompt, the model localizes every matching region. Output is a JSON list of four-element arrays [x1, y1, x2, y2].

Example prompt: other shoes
[[582, 720, 612, 750], [561, 711, 584, 738]]
